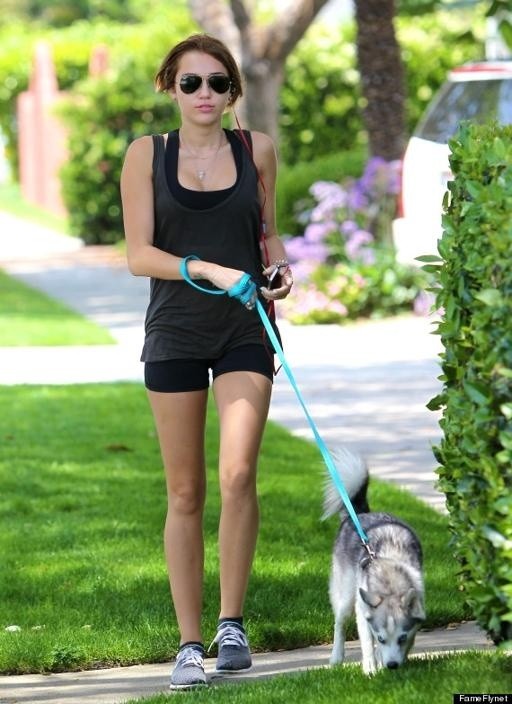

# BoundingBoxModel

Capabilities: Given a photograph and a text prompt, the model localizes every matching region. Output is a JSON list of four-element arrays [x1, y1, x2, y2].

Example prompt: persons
[[119, 33, 294, 692]]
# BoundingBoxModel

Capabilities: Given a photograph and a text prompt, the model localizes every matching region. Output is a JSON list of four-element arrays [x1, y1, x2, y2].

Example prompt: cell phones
[[268, 264, 279, 292]]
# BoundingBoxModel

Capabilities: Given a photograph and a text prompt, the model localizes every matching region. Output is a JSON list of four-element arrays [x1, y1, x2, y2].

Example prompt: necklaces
[[179, 132, 224, 180]]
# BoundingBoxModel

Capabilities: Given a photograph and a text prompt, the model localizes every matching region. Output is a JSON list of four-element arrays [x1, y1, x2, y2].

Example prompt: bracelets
[[273, 259, 289, 263]]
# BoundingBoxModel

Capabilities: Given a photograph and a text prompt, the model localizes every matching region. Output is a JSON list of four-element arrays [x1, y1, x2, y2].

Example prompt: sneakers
[[169, 642, 209, 692], [213, 619, 253, 675]]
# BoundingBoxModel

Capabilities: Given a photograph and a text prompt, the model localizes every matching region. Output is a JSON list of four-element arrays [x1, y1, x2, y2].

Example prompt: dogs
[[317, 444, 430, 681]]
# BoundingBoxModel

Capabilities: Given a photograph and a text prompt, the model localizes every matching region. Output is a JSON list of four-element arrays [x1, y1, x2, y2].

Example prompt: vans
[[389, 58, 511, 292]]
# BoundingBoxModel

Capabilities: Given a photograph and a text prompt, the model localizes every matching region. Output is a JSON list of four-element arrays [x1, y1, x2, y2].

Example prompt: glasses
[[176, 73, 231, 94]]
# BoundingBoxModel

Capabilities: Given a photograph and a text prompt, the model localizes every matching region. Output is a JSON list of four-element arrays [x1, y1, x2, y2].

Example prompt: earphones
[[230, 86, 238, 95]]
